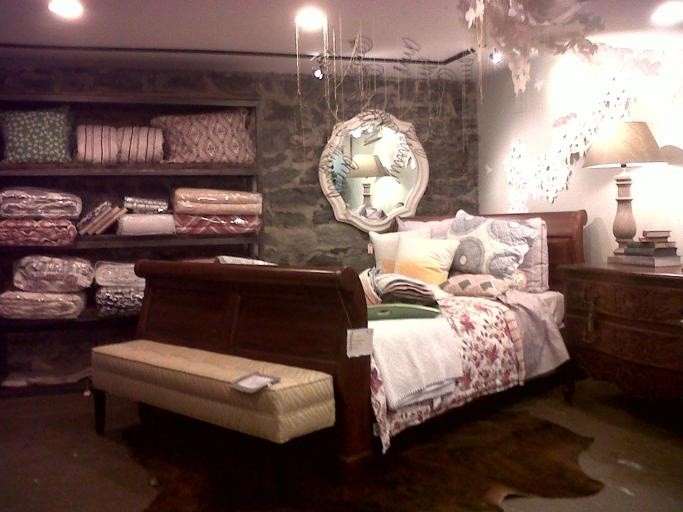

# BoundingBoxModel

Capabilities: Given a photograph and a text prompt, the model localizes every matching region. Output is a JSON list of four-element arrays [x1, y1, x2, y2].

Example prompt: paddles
[[0, 384, 606, 512]]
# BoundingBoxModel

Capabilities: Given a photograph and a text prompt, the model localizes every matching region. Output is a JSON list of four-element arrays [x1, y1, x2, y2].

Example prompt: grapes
[[559, 249, 682, 408]]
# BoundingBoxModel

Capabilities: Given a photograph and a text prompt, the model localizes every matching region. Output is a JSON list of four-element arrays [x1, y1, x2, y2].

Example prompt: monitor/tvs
[[76, 197, 127, 237], [607, 228, 681, 269]]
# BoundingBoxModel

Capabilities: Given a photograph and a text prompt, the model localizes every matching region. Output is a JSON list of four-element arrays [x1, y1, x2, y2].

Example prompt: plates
[[0, 86, 266, 401]]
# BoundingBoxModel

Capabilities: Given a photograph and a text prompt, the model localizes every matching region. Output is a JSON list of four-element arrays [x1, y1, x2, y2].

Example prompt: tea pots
[[315, 107, 434, 239]]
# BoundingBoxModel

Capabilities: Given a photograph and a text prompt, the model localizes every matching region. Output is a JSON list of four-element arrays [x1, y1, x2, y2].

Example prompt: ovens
[[132, 208, 590, 472]]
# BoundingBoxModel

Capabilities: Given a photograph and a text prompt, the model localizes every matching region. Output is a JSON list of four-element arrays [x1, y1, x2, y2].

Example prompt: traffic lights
[[514, 217, 550, 292], [366, 227, 395, 276], [389, 233, 461, 287], [393, 214, 450, 240], [439, 210, 538, 282]]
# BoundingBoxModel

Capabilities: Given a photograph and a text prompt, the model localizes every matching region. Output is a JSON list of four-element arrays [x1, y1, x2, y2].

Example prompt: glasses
[[580, 119, 668, 256]]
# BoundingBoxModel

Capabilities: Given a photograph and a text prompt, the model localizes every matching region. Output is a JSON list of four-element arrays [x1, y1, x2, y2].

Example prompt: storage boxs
[[83, 337, 340, 501]]
